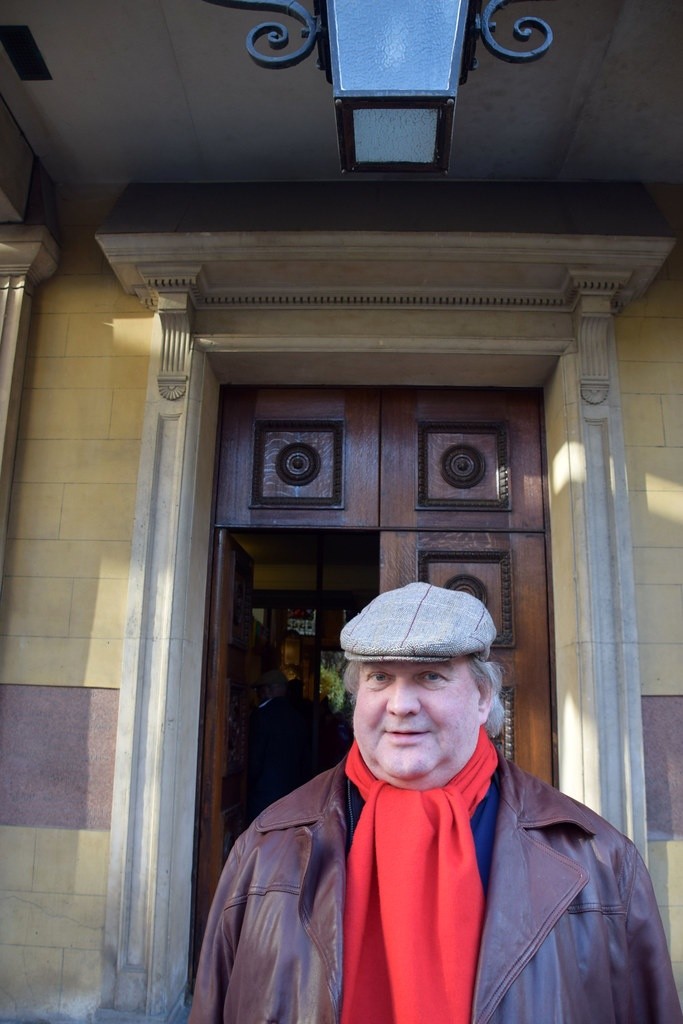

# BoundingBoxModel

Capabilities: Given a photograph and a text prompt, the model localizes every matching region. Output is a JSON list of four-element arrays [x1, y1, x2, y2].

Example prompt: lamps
[[207, 0, 554, 175]]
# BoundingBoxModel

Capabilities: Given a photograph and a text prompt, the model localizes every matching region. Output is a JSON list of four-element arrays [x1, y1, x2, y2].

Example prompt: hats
[[339, 581, 497, 663], [249, 671, 288, 689]]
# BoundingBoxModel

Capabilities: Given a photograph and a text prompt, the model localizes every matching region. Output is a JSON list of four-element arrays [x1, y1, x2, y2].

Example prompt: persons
[[243, 669, 336, 823], [188, 583, 683, 1024]]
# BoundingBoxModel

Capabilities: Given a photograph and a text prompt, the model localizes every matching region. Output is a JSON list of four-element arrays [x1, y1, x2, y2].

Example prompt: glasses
[[253, 686, 279, 692]]
